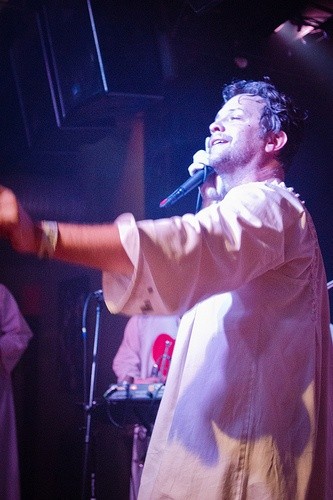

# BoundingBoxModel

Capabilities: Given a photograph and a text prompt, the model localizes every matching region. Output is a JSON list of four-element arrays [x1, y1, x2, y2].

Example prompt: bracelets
[[36, 221, 59, 261]]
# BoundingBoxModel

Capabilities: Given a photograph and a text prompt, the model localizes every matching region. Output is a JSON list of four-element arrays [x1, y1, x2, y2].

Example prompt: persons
[[0, 83, 333, 500], [111, 314, 184, 500], [0, 281, 32, 500]]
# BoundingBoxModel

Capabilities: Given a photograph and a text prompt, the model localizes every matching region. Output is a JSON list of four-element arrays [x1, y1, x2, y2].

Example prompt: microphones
[[92, 290, 104, 300], [159, 165, 215, 208]]
